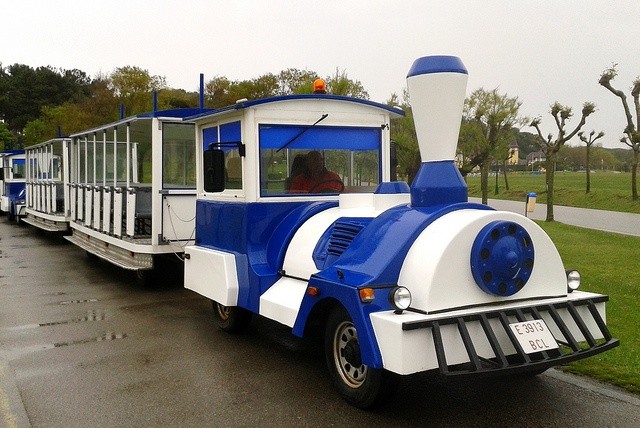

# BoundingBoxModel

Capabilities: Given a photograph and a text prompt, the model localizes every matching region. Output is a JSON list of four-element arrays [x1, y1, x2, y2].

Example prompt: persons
[[288, 151, 341, 193]]
